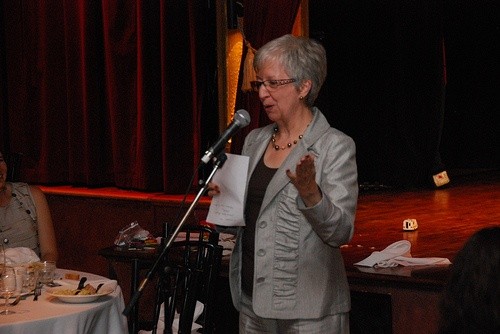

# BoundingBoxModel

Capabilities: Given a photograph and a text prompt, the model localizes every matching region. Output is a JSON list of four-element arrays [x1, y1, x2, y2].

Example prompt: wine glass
[[0, 268, 17, 316]]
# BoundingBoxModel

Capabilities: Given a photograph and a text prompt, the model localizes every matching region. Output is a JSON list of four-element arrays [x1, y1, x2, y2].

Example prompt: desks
[[98, 233, 195, 334], [0, 267, 129, 334]]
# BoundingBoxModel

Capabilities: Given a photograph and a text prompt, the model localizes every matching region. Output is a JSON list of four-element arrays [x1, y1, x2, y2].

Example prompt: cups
[[14, 260, 56, 295], [0, 245, 6, 266]]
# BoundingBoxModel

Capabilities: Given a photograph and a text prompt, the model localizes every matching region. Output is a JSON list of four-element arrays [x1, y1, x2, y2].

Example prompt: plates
[[51, 289, 112, 303]]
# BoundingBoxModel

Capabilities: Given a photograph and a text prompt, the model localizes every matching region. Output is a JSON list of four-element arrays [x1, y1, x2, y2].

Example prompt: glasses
[[250, 78, 297, 92]]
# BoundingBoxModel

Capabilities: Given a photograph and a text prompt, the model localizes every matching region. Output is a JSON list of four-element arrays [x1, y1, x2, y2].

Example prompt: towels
[[355, 239, 452, 269]]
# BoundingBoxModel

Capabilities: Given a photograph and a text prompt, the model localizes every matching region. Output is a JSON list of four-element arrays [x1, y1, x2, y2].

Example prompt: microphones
[[201, 109, 251, 166]]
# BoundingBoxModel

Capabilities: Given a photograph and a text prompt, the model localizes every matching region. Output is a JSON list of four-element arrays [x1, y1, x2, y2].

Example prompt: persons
[[0, 149, 57, 268], [207, 34, 359, 334], [438, 225, 500, 334]]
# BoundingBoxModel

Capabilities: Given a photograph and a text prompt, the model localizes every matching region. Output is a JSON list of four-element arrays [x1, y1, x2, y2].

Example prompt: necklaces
[[272, 128, 303, 150]]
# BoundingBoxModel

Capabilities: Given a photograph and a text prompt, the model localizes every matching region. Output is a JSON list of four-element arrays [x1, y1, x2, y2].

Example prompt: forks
[[10, 289, 41, 306]]
